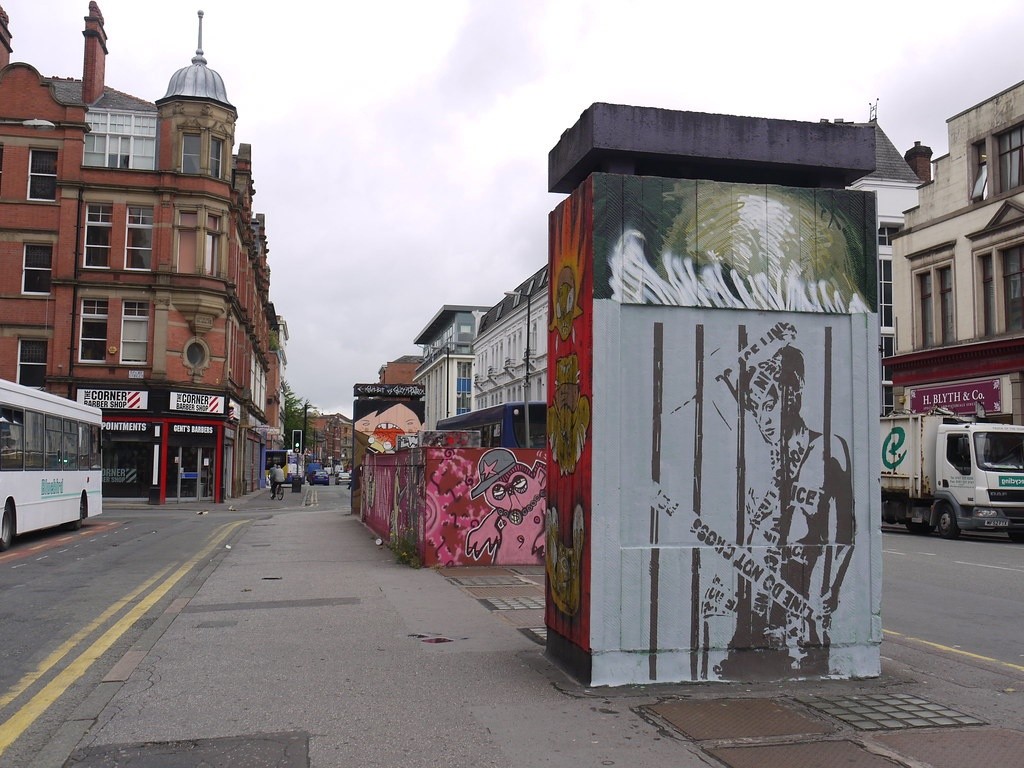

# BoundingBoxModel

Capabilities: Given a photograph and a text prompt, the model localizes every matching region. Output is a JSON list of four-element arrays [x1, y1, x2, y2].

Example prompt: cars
[[309, 470, 330, 486], [335, 472, 352, 485]]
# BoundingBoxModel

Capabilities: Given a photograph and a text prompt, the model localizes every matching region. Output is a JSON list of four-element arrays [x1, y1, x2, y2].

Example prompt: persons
[[268, 464, 285, 499]]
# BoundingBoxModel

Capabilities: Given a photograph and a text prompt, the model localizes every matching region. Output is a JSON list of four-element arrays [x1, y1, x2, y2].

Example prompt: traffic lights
[[291, 429, 303, 453]]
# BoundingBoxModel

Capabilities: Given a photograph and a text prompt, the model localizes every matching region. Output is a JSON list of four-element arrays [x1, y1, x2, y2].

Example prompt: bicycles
[[267, 477, 285, 500]]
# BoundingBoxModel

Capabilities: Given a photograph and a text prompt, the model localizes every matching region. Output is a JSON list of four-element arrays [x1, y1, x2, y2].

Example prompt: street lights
[[313, 427, 319, 463], [503, 291, 533, 385], [430, 345, 450, 418], [302, 404, 319, 454]]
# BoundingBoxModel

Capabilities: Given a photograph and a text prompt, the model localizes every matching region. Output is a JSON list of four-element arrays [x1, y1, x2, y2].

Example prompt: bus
[[435, 400, 547, 449], [265, 449, 306, 486], [0, 377, 105, 551]]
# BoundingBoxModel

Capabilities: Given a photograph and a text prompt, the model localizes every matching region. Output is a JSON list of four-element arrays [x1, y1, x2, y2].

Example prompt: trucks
[[878, 403, 1023, 545]]
